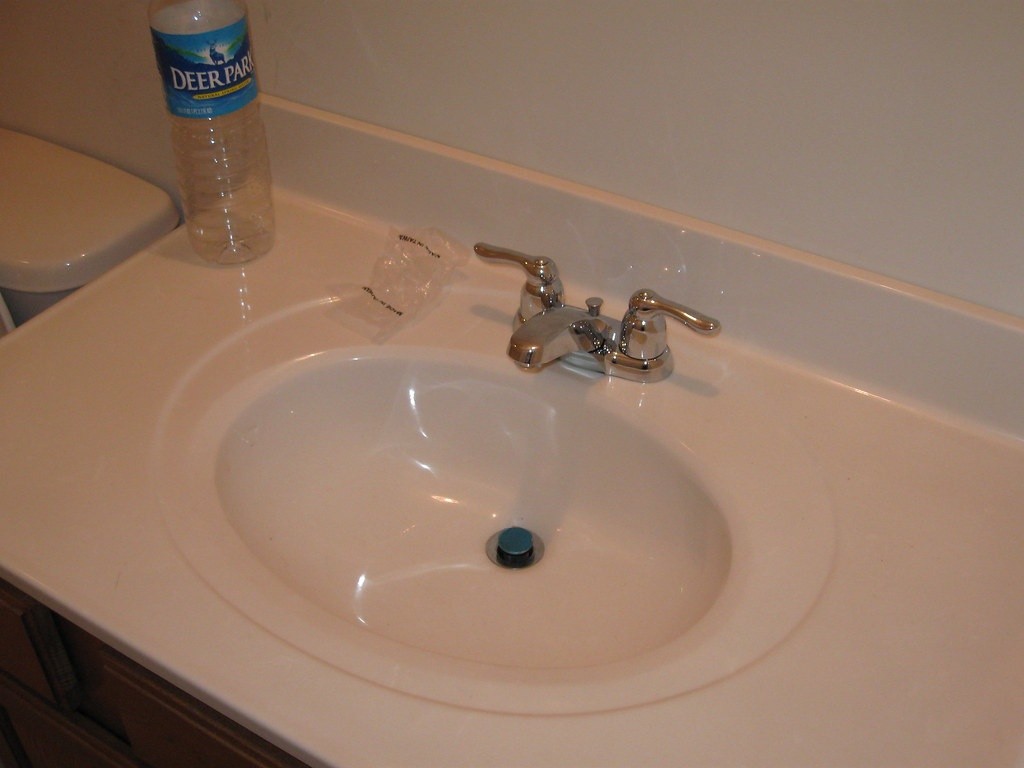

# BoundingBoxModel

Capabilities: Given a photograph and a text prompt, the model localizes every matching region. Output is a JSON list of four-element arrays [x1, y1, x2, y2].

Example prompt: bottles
[[146, 1, 275, 266]]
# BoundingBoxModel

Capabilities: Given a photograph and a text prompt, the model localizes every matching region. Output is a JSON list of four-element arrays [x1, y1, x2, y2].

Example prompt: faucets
[[505, 302, 612, 371]]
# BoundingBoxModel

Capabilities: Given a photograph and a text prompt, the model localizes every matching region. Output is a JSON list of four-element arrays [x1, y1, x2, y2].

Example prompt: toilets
[[0, 121, 184, 337]]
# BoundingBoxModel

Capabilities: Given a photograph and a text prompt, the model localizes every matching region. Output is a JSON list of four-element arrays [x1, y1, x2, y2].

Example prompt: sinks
[[149, 286, 842, 715]]
